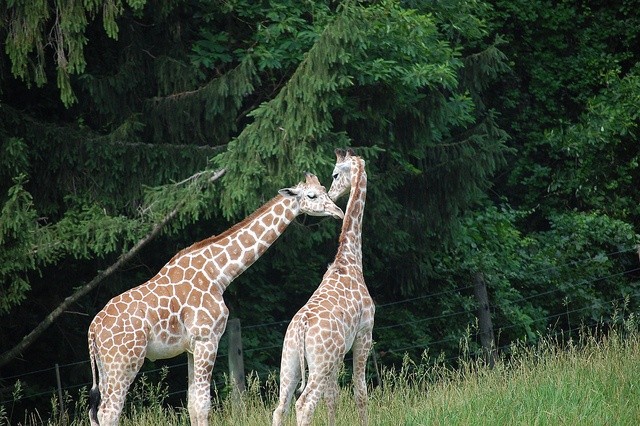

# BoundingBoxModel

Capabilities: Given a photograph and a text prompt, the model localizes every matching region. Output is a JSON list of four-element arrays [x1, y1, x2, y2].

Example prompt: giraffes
[[271, 147, 376, 426], [86, 170, 345, 426]]
